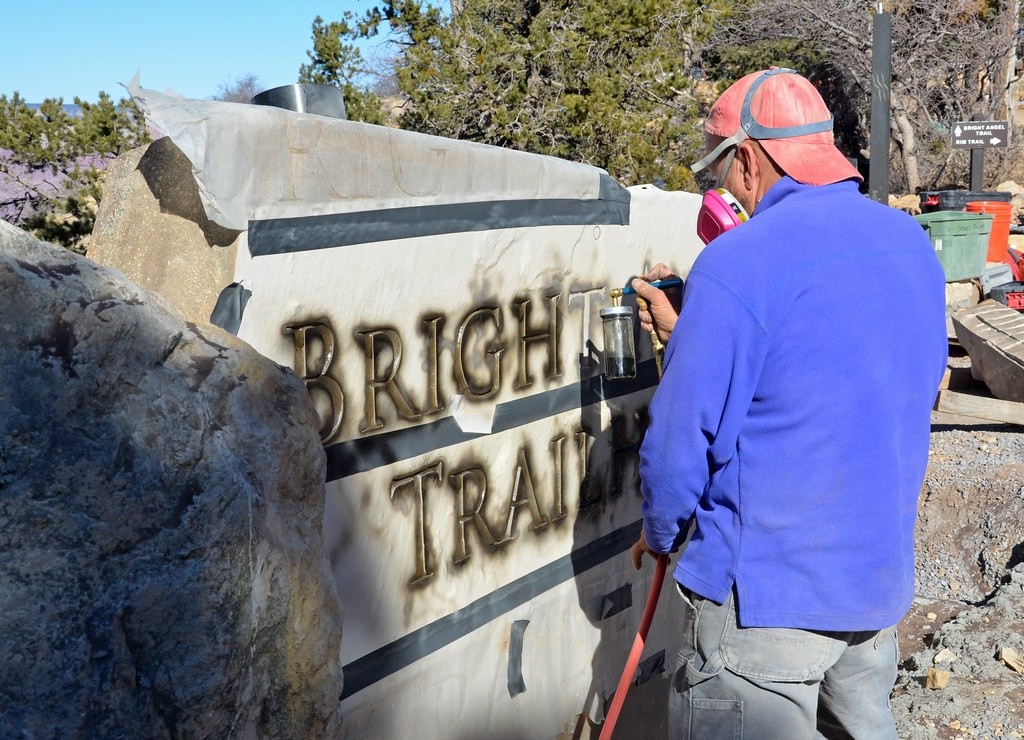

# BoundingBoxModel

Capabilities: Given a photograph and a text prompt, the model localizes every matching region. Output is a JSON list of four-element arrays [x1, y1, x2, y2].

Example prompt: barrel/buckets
[[966, 201, 1012, 262]]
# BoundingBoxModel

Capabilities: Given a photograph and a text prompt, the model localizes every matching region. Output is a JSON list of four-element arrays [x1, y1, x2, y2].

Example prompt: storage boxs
[[911, 210, 996, 280]]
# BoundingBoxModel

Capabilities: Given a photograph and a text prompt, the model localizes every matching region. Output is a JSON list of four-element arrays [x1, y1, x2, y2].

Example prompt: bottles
[[599, 306, 638, 380]]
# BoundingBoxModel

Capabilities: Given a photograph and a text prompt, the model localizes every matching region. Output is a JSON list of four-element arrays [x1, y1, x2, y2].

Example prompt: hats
[[704, 65, 864, 186]]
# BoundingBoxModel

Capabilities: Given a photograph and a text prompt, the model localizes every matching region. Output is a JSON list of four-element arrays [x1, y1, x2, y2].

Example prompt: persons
[[627, 66, 950, 739]]
